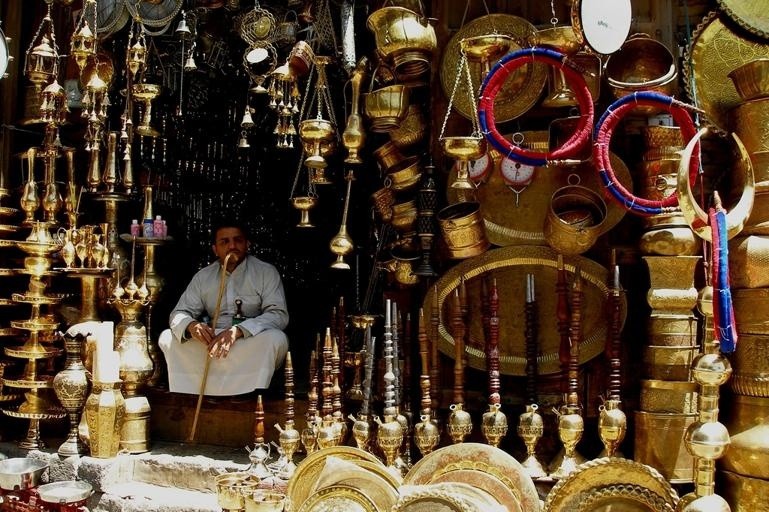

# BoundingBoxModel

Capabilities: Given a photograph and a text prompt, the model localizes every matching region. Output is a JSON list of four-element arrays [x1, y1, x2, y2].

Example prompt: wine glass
[[302, 126, 337, 185], [458, 34, 513, 98], [290, 194, 319, 230], [439, 135, 489, 192], [298, 116, 337, 170], [526, 24, 585, 108]]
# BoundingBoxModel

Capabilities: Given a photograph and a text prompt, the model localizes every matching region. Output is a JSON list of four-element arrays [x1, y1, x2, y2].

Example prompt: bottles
[[129, 214, 167, 240]]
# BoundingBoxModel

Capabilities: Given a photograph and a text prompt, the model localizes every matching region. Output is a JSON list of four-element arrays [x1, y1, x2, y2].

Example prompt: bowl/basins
[[0, 456, 52, 492]]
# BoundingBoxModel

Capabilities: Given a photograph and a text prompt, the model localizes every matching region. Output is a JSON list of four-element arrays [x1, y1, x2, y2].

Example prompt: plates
[[35, 481, 94, 504]]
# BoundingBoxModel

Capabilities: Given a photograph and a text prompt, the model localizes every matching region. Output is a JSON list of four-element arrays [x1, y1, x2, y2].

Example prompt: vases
[[52, 328, 93, 458]]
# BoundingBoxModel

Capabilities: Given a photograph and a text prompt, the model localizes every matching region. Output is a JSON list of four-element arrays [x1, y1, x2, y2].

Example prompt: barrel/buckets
[[543, 173, 607, 255], [436, 194, 490, 258]]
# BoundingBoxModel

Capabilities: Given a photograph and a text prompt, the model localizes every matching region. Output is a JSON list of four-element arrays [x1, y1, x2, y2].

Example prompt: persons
[[158, 220, 290, 396]]
